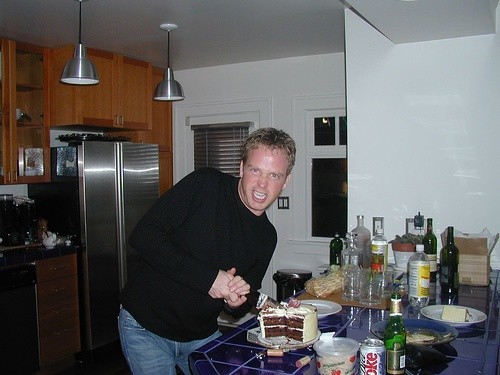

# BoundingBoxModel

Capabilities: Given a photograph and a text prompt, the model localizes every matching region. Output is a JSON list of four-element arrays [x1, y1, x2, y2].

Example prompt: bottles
[[407, 244, 430, 304], [383, 293, 406, 375], [422, 218, 437, 283], [351, 215, 371, 270], [343, 232, 364, 269], [370, 229, 388, 273], [440, 226, 459, 294], [329, 234, 343, 267]]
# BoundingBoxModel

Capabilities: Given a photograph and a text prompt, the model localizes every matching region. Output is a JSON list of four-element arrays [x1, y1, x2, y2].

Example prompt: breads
[[305, 271, 347, 298]]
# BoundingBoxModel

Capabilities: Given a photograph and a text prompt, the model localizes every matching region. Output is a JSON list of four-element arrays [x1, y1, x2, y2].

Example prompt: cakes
[[258, 298, 318, 342]]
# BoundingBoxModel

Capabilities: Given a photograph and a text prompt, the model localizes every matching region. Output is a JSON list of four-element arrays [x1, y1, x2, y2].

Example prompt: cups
[[16, 109, 22, 120], [341, 267, 382, 306]]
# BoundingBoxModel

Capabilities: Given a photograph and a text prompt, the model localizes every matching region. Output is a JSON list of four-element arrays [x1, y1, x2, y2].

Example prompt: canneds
[[359, 338, 387, 375]]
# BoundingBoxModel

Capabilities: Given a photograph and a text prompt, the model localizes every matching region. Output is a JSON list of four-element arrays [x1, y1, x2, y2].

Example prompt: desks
[[188, 268, 500, 375]]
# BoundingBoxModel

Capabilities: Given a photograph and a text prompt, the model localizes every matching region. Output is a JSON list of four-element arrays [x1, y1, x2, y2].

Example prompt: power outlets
[[372, 217, 384, 236], [277, 196, 290, 210]]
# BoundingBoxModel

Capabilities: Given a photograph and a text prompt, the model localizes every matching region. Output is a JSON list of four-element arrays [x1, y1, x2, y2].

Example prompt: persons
[[118, 128, 295, 375]]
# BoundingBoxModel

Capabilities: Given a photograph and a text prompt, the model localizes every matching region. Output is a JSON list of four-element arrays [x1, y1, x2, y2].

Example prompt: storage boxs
[[440, 225, 500, 287]]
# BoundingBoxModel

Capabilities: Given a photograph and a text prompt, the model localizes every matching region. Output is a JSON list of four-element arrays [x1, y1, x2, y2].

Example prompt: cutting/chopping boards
[[296, 290, 404, 309]]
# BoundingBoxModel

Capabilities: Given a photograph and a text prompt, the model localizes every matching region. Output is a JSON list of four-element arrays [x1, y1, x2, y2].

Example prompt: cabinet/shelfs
[[0, 31, 173, 375]]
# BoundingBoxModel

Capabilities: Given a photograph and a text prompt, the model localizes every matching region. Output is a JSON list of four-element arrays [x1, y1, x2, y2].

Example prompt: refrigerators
[[78, 141, 160, 349]]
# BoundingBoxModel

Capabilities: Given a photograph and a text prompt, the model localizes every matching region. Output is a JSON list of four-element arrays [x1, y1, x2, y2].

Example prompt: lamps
[[153, 24, 186, 101], [60, 0, 100, 85]]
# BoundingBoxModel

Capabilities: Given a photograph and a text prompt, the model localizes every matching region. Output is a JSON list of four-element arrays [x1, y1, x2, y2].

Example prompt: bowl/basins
[[370, 318, 459, 345], [420, 305, 487, 328], [313, 337, 359, 375], [43, 242, 56, 249]]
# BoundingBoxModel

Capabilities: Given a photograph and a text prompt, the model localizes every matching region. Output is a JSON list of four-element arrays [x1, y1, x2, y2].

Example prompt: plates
[[299, 299, 342, 320]]
